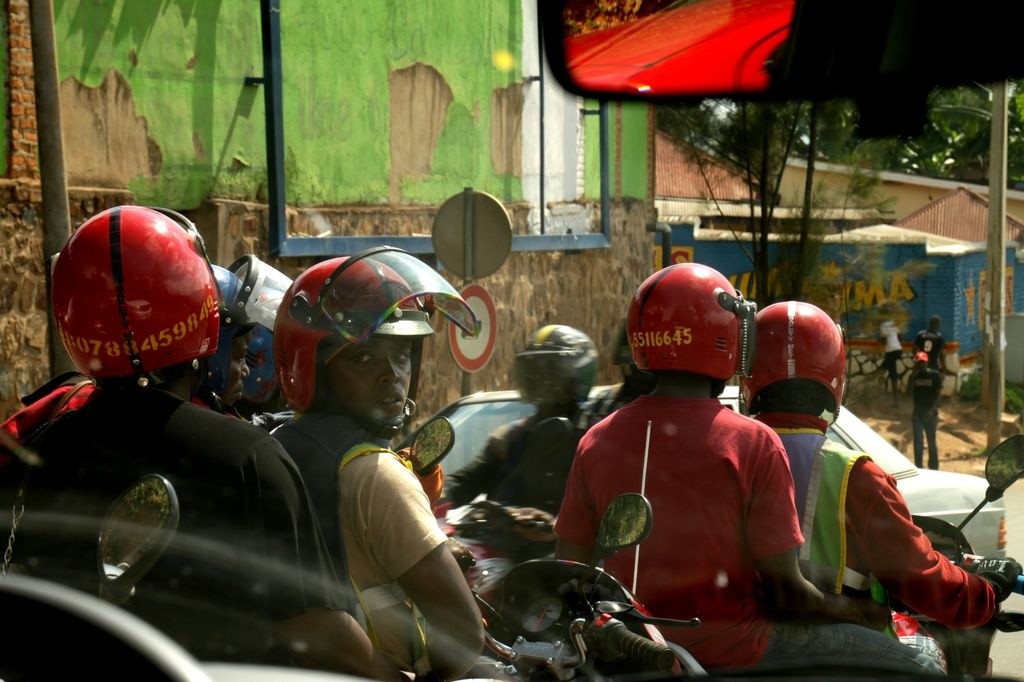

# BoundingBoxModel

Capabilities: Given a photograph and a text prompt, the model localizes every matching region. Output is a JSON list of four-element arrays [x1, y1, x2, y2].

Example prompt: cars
[[399, 390, 1007, 582]]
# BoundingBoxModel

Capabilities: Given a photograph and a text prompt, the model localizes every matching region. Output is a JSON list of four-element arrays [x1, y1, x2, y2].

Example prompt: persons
[[906, 353, 942, 471], [912, 316, 946, 383], [876, 307, 903, 408], [0, 205, 637, 682], [741, 299, 1024, 645], [554, 261, 946, 682]]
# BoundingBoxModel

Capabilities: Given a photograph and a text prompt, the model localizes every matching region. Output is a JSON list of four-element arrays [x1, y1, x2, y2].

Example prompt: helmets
[[51, 204, 222, 381], [626, 262, 742, 382], [914, 351, 929, 362], [272, 256, 436, 414], [737, 301, 847, 413], [515, 323, 599, 403], [177, 260, 259, 404]]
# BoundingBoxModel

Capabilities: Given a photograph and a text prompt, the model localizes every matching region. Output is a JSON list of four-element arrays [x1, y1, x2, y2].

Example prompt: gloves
[[975, 556, 1022, 601]]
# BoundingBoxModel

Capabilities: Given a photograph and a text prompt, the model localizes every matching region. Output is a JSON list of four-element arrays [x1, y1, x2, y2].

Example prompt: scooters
[[889, 435, 1024, 680], [400, 416, 562, 590], [456, 493, 707, 680]]
